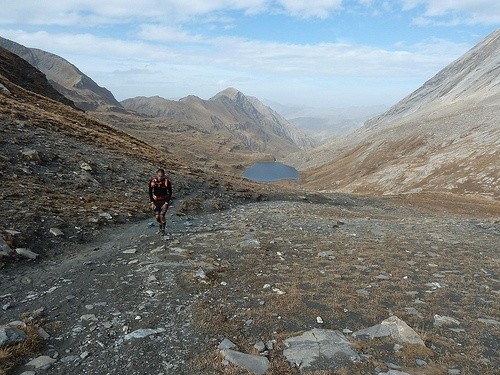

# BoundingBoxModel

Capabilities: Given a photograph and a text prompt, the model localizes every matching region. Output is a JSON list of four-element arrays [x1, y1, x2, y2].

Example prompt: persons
[[149, 169, 173, 234]]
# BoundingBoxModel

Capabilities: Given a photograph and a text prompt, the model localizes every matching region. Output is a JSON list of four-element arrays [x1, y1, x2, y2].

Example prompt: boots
[[157, 222, 162, 234], [161, 222, 166, 236]]
